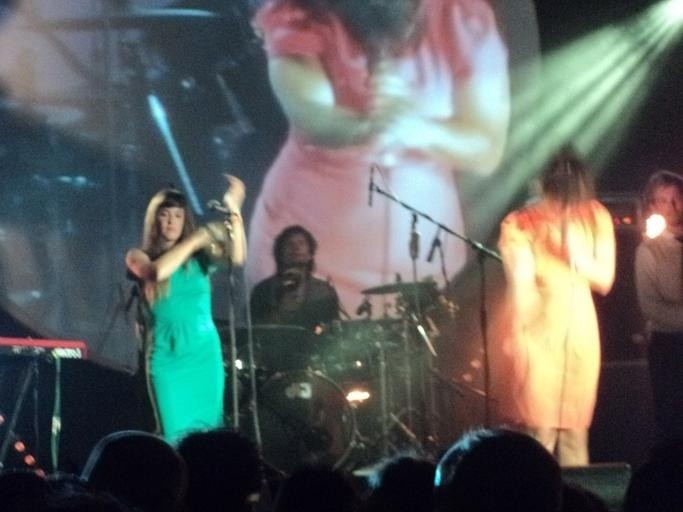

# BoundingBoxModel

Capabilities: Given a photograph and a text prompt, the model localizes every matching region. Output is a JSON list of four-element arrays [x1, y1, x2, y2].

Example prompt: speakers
[[593, 193, 649, 358]]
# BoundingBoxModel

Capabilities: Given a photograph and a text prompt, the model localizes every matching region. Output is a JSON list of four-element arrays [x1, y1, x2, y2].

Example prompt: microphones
[[207, 200, 234, 216], [427, 224, 445, 262], [366, 164, 376, 208]]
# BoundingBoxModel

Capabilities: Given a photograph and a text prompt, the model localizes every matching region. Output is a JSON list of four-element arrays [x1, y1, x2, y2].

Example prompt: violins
[[192, 218, 234, 268]]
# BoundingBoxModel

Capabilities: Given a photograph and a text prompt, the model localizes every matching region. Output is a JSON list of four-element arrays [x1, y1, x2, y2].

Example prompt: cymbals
[[361, 281, 436, 294]]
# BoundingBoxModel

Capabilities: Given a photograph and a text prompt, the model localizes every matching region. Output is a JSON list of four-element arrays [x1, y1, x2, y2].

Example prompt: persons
[[633, 169, 683, 430], [249, 225, 339, 323], [248, 1, 512, 320], [497, 144, 618, 467], [1, 429, 682, 511], [125, 173, 248, 450]]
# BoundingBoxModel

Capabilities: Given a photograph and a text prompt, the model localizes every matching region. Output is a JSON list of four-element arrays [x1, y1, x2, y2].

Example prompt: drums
[[377, 318, 431, 369], [249, 367, 357, 477], [320, 319, 387, 376], [227, 322, 314, 379]]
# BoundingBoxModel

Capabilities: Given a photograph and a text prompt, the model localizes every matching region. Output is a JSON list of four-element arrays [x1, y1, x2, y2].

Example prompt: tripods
[[353, 274, 459, 466]]
